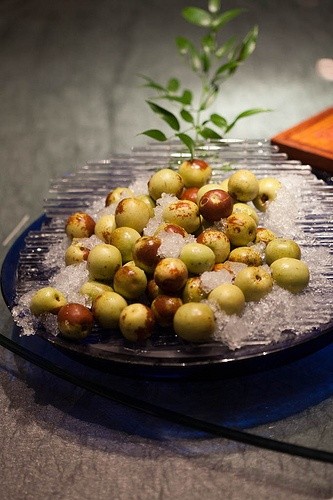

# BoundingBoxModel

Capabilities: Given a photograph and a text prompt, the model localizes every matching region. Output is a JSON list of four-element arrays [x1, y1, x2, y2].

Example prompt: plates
[[1, 165, 333, 382]]
